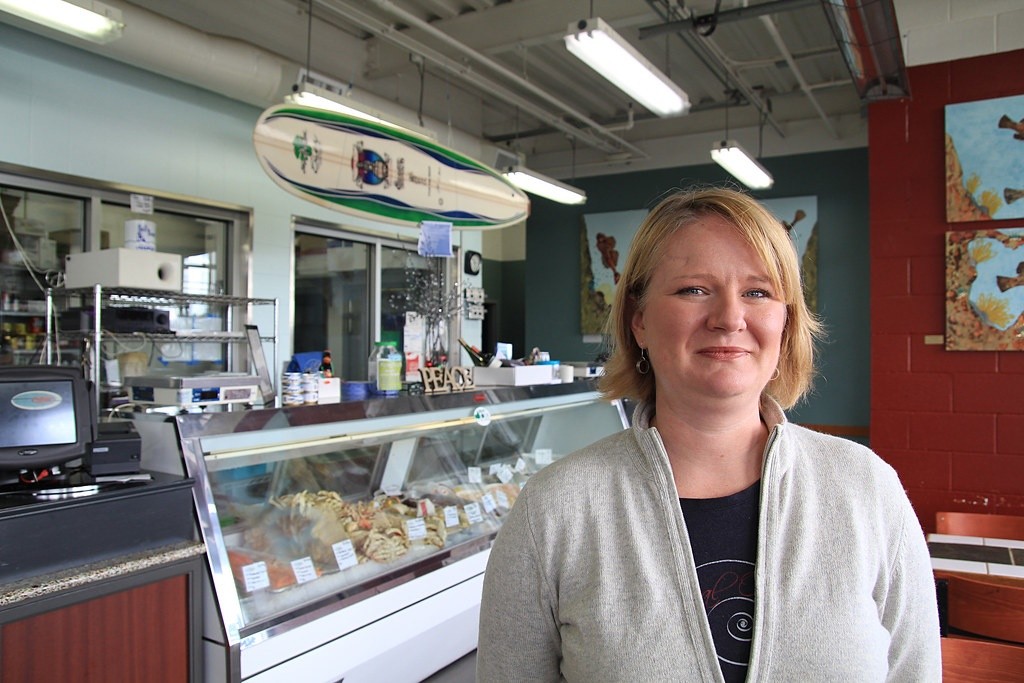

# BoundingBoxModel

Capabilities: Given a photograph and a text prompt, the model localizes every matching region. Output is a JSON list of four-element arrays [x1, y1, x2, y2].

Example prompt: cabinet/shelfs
[[45, 284, 279, 425]]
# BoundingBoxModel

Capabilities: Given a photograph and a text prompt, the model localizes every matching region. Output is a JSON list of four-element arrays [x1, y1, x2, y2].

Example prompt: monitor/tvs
[[0, 366, 101, 473]]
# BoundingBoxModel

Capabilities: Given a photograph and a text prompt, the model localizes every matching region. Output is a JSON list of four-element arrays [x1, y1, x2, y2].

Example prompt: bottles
[[458, 337, 513, 367]]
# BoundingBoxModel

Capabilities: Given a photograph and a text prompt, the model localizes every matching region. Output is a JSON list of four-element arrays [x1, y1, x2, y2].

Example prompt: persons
[[476, 187, 943, 683]]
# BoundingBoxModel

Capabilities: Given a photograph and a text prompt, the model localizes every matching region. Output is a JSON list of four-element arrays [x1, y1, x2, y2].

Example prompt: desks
[[927, 533, 1024, 590]]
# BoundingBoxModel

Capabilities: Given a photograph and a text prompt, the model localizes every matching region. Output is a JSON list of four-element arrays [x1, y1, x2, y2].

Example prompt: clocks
[[466, 251, 482, 274]]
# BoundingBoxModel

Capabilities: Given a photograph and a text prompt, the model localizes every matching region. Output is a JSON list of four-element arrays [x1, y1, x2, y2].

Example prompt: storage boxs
[[473, 366, 553, 386], [574, 367, 604, 379]]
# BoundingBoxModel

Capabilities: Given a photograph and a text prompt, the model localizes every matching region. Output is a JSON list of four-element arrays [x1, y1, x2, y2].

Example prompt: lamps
[[711, 141, 773, 190], [503, 166, 587, 207], [287, 80, 437, 144], [564, 17, 690, 120], [0, 0, 125, 47]]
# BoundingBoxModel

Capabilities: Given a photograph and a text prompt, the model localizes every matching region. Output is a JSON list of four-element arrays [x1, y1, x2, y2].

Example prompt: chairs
[[936, 511, 1024, 683]]
